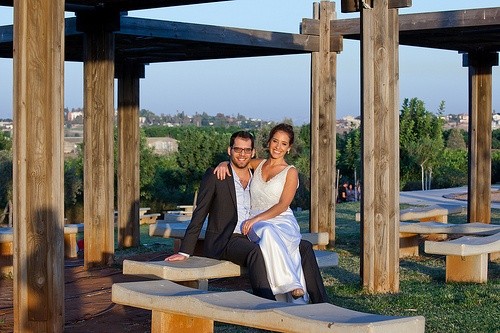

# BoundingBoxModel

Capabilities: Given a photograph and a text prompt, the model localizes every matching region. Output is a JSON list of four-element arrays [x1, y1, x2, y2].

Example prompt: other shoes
[[292, 289, 305, 298]]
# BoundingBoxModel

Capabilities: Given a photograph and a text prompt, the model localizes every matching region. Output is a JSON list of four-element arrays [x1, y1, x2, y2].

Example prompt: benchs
[[114, 213, 161, 227], [355, 202, 500, 284], [0, 225, 79, 258], [150, 223, 339, 255], [122, 249, 340, 294], [167, 211, 193, 218], [111, 280, 425, 333]]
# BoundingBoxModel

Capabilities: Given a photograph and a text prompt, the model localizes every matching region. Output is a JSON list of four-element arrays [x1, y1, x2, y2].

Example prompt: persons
[[213, 123, 309, 304], [164, 131, 329, 304], [338, 182, 355, 204]]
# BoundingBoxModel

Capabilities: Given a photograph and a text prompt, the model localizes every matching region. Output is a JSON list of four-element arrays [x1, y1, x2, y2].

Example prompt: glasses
[[231, 147, 253, 153]]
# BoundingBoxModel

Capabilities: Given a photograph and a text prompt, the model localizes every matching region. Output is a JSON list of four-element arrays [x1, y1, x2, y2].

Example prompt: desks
[[177, 205, 197, 222], [114, 208, 151, 215]]
